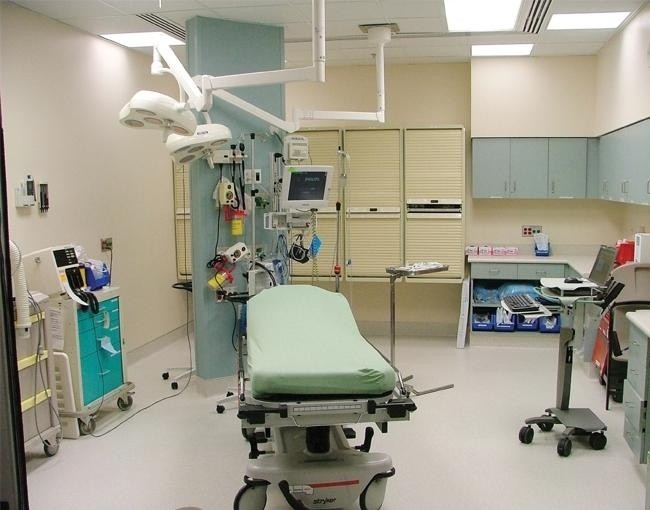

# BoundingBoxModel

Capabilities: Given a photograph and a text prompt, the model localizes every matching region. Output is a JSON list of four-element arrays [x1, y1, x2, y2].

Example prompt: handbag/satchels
[[287, 234, 309, 264]]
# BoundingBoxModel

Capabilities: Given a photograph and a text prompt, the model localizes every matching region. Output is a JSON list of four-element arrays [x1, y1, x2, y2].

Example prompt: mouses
[[565, 277, 580, 283]]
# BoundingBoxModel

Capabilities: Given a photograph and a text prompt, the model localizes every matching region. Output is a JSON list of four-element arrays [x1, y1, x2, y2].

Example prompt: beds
[[221, 284, 418, 509]]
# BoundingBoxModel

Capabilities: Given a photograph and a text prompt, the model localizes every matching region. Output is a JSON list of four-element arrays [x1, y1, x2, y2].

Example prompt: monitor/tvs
[[282, 165, 331, 208]]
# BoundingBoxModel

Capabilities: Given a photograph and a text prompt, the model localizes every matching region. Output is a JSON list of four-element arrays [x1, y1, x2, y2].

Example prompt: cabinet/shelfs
[[12, 308, 65, 466], [617, 309, 649, 469], [469, 113, 650, 206], [49, 283, 135, 436]]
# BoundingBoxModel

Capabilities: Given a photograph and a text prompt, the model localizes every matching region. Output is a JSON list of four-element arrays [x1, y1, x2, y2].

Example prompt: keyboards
[[503, 295, 540, 311]]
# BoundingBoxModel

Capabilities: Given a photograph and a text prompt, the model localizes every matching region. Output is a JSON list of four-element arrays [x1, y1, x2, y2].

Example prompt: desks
[[563, 252, 607, 364], [464, 246, 569, 332]]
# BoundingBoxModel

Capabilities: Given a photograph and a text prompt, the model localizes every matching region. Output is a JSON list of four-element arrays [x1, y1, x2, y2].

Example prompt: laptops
[[551, 245, 617, 296]]
